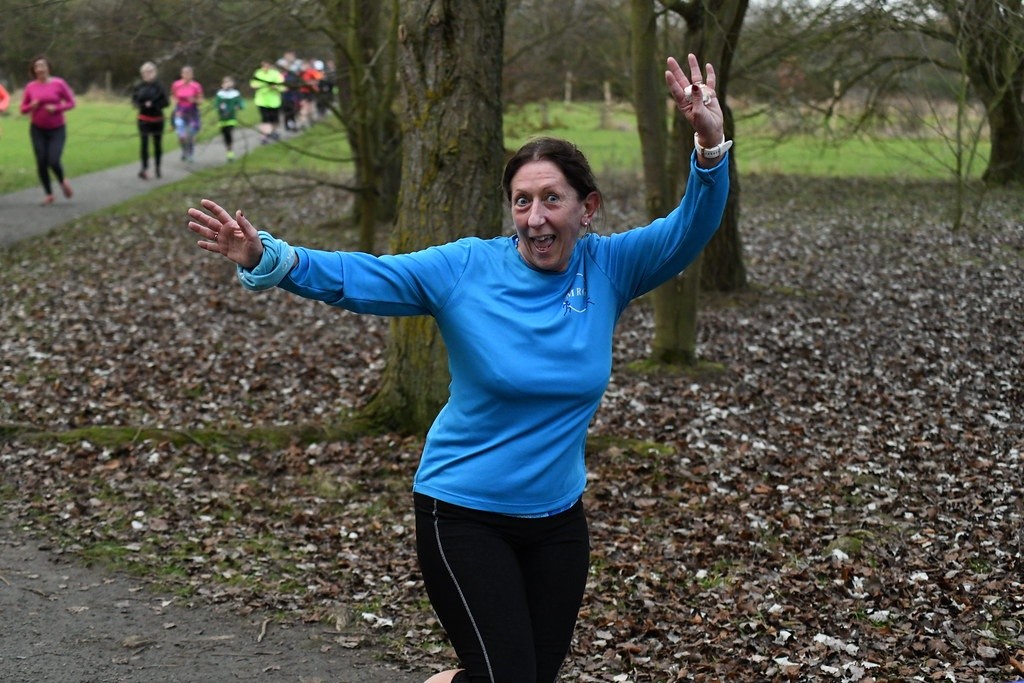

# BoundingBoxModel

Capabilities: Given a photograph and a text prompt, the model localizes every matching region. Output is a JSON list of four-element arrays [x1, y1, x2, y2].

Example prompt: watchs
[[693, 130, 733, 158]]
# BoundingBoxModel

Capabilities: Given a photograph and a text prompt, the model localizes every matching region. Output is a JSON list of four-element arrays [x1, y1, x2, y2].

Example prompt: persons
[[20, 55, 79, 206], [169, 61, 204, 163], [247, 44, 342, 142], [183, 53, 740, 682], [0, 80, 12, 117], [130, 59, 172, 179], [202, 72, 246, 164]]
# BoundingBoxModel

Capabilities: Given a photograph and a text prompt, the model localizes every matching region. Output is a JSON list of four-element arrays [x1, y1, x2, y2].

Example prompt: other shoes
[[61, 176, 74, 199], [136, 168, 149, 180], [226, 152, 234, 161], [180, 155, 195, 167], [153, 167, 161, 179], [39, 195, 56, 206]]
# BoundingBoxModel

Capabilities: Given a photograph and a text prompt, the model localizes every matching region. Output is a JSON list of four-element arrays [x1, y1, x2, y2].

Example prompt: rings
[[694, 81, 704, 85], [213, 231, 219, 242]]
